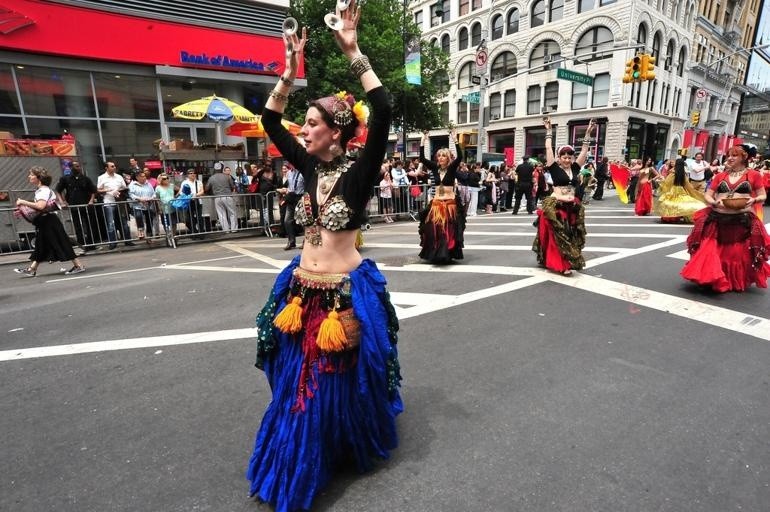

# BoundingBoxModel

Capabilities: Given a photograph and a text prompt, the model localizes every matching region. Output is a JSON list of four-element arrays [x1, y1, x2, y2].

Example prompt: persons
[[680, 145, 769, 293], [608, 152, 769, 224], [245, 0, 404, 512], [375, 154, 609, 223], [56, 159, 304, 251], [13, 165, 85, 277], [416, 125, 468, 265], [531, 118, 596, 275]]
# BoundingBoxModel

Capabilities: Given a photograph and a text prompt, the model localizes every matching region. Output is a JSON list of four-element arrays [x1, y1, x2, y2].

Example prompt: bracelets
[[269, 91, 289, 106], [350, 56, 373, 80], [280, 77, 294, 88]]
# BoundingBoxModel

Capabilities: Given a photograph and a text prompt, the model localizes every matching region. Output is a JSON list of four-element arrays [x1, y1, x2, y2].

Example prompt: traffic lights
[[622, 58, 633, 83], [628, 56, 645, 80], [648, 53, 661, 79]]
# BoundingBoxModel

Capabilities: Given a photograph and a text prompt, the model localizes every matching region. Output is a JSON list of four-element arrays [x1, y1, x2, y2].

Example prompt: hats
[[522, 155, 529, 159], [214, 163, 222, 170]]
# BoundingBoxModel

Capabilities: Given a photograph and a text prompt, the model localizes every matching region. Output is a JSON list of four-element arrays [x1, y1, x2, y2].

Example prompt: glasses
[[163, 177, 169, 180]]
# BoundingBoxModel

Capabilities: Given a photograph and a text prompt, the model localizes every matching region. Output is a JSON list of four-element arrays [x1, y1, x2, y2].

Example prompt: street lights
[[696, 36, 770, 165]]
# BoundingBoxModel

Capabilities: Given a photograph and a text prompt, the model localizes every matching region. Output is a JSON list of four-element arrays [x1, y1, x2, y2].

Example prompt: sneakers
[[384, 216, 394, 223], [486, 204, 507, 213], [65, 266, 85, 275], [14, 268, 35, 277]]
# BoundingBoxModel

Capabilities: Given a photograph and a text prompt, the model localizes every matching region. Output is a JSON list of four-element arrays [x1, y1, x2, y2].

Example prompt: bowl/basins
[[722, 198, 750, 210]]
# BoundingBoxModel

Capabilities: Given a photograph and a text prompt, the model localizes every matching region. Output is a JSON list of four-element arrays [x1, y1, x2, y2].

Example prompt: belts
[[691, 179, 704, 182]]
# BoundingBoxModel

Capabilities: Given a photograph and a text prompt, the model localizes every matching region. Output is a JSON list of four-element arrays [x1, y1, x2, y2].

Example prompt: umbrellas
[[172, 93, 259, 162], [225, 115, 303, 166]]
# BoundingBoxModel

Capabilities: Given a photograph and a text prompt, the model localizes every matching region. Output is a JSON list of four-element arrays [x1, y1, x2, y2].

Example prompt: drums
[[18, 199, 61, 220]]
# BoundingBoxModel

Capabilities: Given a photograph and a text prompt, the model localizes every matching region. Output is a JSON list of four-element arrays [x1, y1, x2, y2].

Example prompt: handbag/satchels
[[249, 181, 259, 193], [188, 199, 200, 211], [705, 169, 713, 180]]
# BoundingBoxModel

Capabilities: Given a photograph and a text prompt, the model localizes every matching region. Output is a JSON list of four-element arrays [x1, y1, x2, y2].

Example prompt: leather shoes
[[109, 245, 116, 249], [285, 242, 295, 250], [125, 243, 134, 246]]
